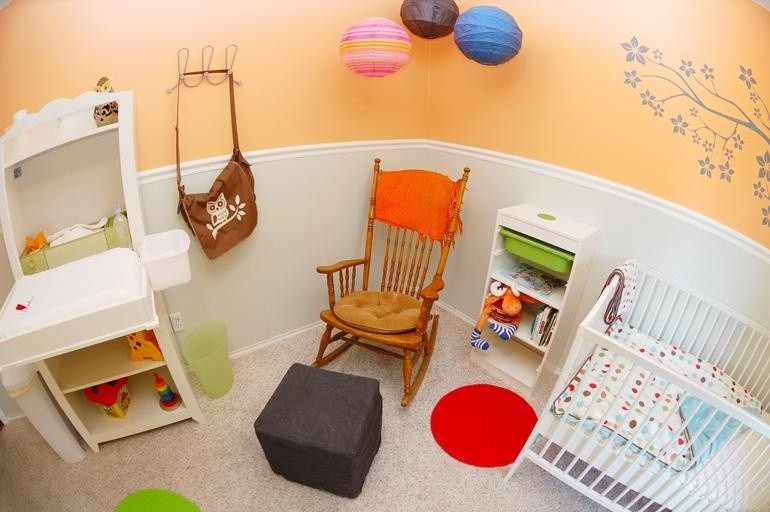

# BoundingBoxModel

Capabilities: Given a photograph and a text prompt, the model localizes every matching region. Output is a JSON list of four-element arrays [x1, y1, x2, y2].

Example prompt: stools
[[254, 363, 383, 498]]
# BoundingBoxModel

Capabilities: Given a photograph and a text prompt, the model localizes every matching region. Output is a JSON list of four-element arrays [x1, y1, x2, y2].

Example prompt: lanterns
[[338, 16, 415, 80], [452, 5, 524, 67], [400, 0, 459, 40]]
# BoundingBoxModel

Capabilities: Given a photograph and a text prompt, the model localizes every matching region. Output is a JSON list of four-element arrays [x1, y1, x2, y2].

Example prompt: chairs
[[310, 159, 471, 407]]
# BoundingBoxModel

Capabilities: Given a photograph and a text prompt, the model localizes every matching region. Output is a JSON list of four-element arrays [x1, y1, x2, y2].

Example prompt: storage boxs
[[499, 228, 575, 275], [43, 221, 109, 270], [104, 209, 134, 249], [19, 242, 49, 275]]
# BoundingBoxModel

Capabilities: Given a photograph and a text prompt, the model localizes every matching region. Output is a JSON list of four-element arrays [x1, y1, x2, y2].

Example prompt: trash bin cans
[[181, 321, 233, 399]]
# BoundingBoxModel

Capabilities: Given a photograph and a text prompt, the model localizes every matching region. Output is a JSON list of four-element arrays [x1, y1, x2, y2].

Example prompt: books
[[501, 262, 569, 298], [528, 306, 559, 348]]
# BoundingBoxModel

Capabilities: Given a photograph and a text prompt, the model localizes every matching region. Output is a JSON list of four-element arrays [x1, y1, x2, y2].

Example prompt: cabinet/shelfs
[[473, 204, 599, 400], [0, 90, 205, 454]]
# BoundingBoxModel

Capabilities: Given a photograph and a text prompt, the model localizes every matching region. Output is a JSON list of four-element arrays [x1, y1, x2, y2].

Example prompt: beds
[[506, 260, 770, 512]]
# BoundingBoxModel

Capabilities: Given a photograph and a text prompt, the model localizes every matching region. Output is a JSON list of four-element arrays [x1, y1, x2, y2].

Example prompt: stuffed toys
[[470, 281, 540, 351]]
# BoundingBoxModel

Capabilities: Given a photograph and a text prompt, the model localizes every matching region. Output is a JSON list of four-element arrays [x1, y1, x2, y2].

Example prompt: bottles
[[113, 207, 133, 252]]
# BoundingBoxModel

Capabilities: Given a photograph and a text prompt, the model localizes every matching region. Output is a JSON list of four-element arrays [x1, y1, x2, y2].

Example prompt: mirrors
[[0, 90, 145, 278]]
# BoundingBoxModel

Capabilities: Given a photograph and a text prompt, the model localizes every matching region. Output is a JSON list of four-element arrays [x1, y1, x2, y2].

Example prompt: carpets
[[115, 489, 202, 512], [431, 384, 538, 468]]
